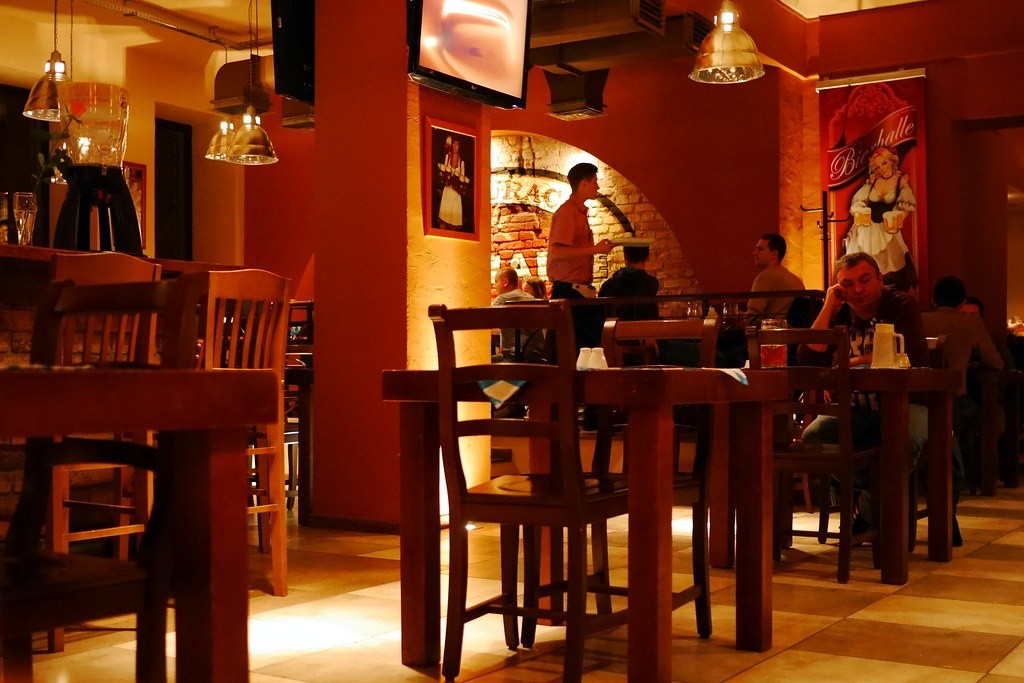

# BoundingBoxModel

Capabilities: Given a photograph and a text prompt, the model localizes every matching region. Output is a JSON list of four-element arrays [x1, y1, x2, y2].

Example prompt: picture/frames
[[122, 160, 147, 249], [425, 115, 482, 240]]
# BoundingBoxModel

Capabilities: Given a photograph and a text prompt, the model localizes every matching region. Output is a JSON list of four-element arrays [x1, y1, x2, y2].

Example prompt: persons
[[723, 233, 805, 329], [796, 251, 928, 543], [598, 246, 659, 366], [547, 163, 621, 433], [921, 276, 1020, 496], [491, 267, 550, 363], [439, 138, 466, 230]]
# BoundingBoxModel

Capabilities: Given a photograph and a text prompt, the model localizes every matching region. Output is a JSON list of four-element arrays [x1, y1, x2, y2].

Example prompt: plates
[[607, 238, 654, 247]]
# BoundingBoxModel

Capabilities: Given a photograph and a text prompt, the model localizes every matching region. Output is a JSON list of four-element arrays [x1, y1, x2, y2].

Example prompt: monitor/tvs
[[405, 0, 533, 108], [270, 0, 316, 104]]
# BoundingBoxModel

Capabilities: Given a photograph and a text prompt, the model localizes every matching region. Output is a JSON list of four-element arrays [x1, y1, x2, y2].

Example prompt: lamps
[[225, 2, 278, 166], [689, 0, 766, 84], [23, 0, 71, 121], [205, 45, 236, 159]]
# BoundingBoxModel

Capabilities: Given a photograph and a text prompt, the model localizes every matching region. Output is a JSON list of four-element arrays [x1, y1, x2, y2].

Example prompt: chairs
[[22, 251, 163, 652], [120, 266, 293, 596], [426, 301, 960, 683]]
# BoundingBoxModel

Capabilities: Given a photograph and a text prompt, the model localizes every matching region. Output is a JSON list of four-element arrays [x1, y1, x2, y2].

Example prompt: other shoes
[[493, 407, 509, 418], [851, 502, 868, 547], [583, 420, 623, 433]]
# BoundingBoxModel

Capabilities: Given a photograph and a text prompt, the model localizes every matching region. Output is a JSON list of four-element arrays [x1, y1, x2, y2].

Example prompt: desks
[[382, 368, 795, 682], [720, 368, 960, 583], [1, 369, 278, 682]]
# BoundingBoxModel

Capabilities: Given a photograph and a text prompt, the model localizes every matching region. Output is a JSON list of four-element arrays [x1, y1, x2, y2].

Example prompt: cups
[[760, 319, 787, 368], [688, 301, 698, 316], [575, 347, 609, 368], [0, 192, 9, 245], [870, 324, 904, 368], [723, 302, 739, 315]]
